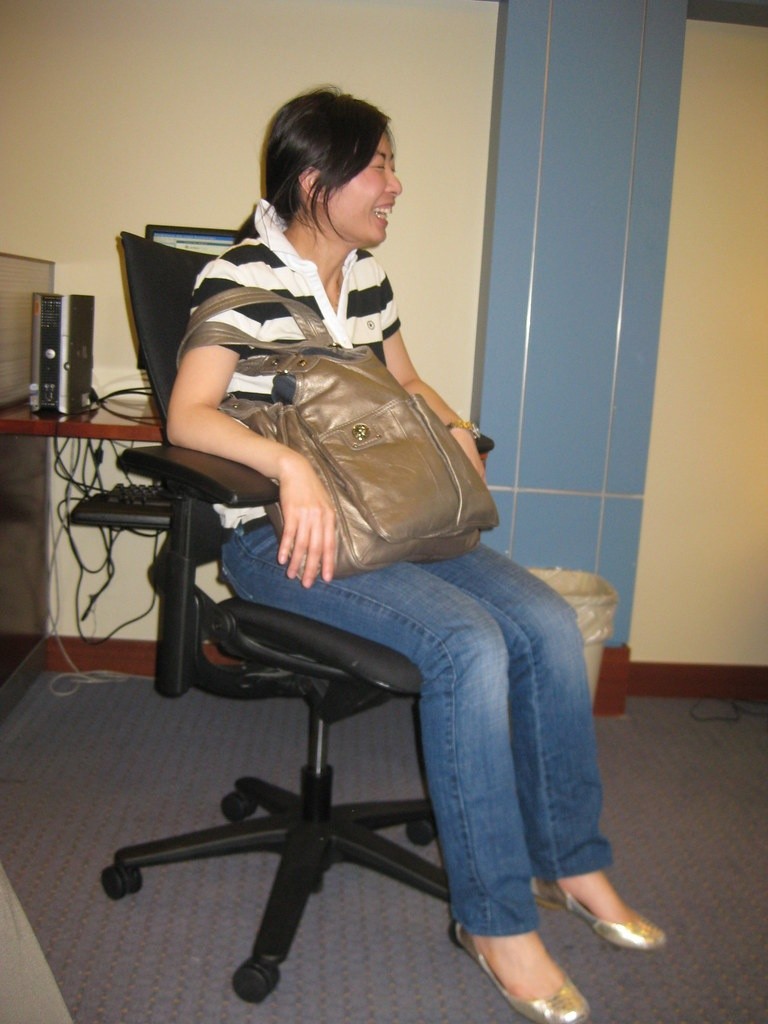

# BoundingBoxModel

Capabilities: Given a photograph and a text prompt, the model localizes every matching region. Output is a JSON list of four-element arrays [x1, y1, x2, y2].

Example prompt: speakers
[[28, 292, 94, 416]]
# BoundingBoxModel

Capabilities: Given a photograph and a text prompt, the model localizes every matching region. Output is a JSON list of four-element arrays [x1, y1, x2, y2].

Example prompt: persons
[[167, 83, 667, 1023]]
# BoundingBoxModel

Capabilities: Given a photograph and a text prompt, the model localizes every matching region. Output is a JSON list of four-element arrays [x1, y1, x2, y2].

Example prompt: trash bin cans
[[529, 569, 619, 712]]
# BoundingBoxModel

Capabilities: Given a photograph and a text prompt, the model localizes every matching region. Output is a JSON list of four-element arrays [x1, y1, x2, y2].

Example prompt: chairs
[[98, 229, 448, 1004]]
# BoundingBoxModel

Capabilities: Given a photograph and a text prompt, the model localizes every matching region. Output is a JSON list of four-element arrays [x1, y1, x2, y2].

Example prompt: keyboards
[[71, 481, 172, 525]]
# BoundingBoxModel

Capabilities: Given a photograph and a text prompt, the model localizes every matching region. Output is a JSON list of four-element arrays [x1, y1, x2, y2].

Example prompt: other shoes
[[530, 875, 668, 951], [455, 920, 590, 1024]]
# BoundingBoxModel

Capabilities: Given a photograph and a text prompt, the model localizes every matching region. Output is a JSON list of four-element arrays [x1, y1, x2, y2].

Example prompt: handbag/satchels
[[175, 287, 500, 579]]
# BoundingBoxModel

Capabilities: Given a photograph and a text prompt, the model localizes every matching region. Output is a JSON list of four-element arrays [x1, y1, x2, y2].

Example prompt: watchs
[[447, 421, 481, 439]]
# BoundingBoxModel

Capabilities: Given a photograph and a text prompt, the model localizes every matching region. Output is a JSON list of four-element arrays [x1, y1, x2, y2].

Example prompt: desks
[[0, 392, 163, 442]]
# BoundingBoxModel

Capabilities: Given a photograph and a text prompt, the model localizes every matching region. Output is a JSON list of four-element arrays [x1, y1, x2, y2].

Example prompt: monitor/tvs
[[136, 226, 239, 369]]
